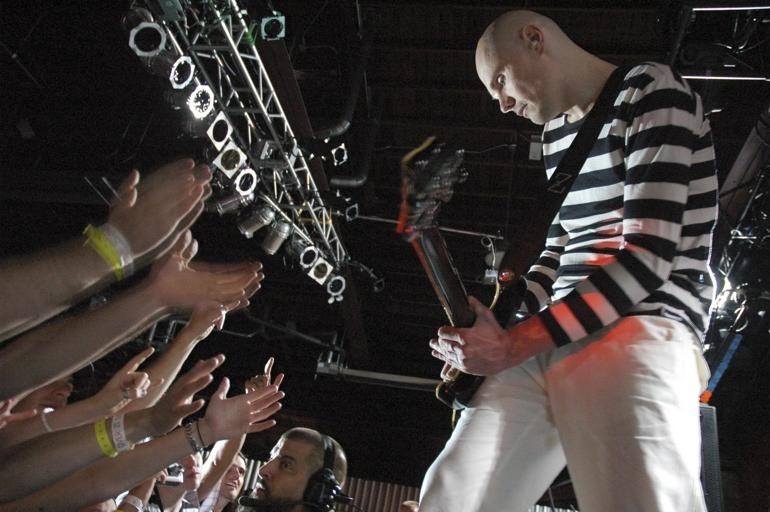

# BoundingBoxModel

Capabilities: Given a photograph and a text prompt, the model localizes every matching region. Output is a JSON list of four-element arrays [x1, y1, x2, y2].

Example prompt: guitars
[[395, 137, 525, 427]]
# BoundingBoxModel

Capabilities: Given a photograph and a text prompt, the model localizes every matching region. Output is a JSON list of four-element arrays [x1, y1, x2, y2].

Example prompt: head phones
[[305, 434, 340, 512]]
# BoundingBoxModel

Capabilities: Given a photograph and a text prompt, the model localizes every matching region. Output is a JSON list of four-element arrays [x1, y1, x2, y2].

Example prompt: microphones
[[238, 496, 322, 512]]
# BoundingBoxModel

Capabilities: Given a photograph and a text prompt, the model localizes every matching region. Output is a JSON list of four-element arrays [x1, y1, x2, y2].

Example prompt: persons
[[0, 154, 284, 512], [416, 10, 719, 510], [241, 427, 349, 510]]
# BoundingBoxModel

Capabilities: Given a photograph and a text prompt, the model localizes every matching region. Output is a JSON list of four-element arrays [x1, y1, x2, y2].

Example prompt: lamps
[[118, 1, 358, 300]]
